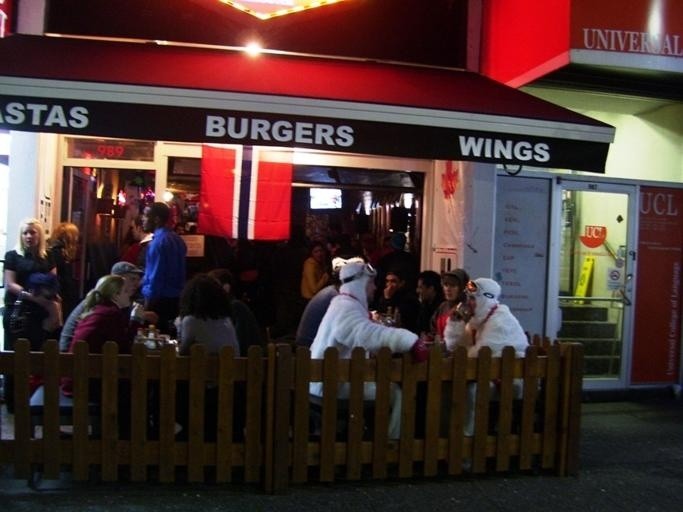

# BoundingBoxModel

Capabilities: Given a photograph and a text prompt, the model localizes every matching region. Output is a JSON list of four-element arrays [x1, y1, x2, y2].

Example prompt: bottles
[[145, 324, 156, 348], [135, 328, 144, 343]]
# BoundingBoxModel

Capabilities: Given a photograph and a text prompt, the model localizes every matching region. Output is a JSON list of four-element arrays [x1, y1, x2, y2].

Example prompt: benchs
[[305, 394, 392, 482], [31, 384, 99, 480]]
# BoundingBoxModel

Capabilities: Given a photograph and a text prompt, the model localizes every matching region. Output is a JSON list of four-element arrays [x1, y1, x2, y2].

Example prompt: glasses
[[342, 264, 377, 282], [465, 280, 495, 299]]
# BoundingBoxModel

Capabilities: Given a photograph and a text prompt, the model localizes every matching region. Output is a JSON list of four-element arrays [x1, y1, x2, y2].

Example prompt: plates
[[422, 340, 442, 344]]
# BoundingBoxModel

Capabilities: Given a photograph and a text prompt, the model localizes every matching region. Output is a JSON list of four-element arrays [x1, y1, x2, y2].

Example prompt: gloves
[[412, 338, 429, 364]]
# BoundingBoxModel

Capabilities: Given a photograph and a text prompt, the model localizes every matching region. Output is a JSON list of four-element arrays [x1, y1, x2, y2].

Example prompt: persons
[[300, 211, 544, 482], [1, 200, 243, 481]]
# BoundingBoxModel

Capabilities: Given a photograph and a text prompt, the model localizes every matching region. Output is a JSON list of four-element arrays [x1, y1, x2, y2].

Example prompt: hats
[[112, 262, 145, 277], [446, 269, 469, 290], [391, 232, 406, 249], [29, 273, 62, 295]]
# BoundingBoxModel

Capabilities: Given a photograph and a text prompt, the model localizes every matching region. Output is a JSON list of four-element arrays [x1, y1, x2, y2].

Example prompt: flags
[[245, 149, 293, 241], [195, 142, 243, 240]]
